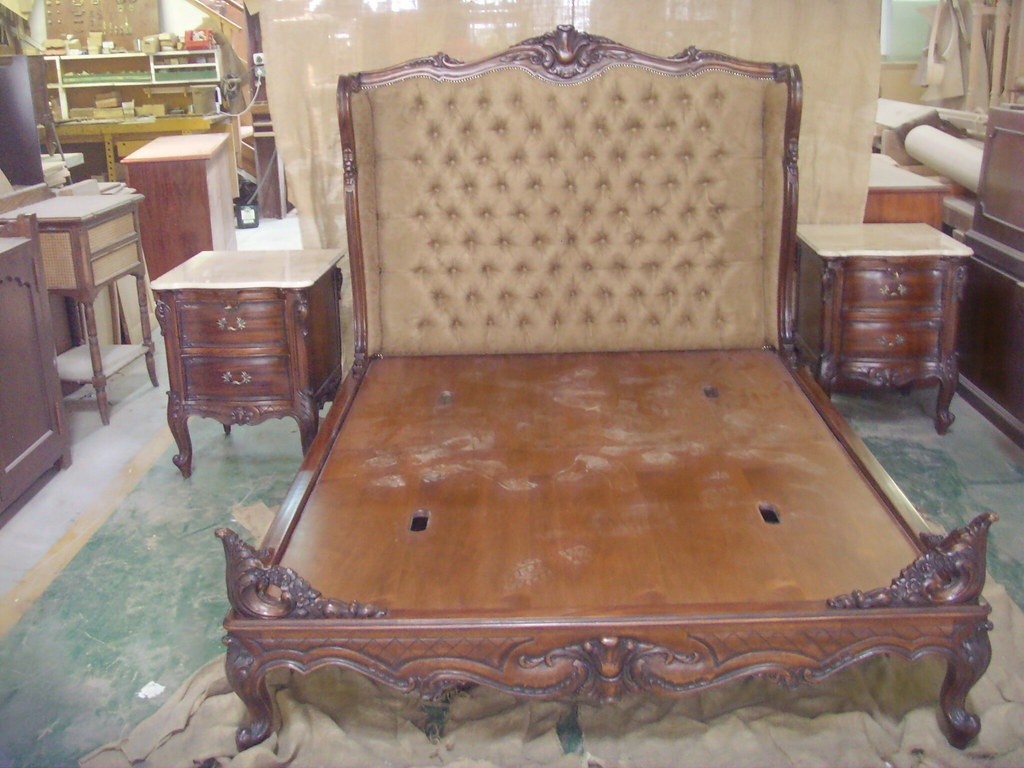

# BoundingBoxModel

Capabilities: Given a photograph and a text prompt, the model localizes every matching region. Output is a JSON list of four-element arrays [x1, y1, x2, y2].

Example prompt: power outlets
[[254, 66, 265, 77], [253, 53, 264, 65]]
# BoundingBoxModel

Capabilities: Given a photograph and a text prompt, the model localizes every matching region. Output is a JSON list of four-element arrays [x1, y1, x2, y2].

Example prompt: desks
[[865, 152, 949, 236], [0, 193, 160, 426], [121, 132, 232, 272]]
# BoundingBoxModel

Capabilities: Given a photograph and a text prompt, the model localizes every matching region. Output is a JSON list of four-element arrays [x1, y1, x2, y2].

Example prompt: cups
[[122, 102, 134, 120]]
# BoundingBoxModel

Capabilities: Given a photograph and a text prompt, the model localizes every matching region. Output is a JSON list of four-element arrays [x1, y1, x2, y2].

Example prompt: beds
[[216, 25, 998, 753]]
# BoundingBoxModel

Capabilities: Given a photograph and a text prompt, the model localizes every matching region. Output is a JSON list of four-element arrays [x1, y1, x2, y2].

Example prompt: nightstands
[[151, 249, 347, 478], [791, 221, 975, 436]]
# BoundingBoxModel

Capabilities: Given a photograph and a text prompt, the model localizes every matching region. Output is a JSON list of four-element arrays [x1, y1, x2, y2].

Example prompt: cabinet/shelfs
[[0, 213, 71, 518], [945, 105, 1024, 447], [46, 48, 225, 143]]
